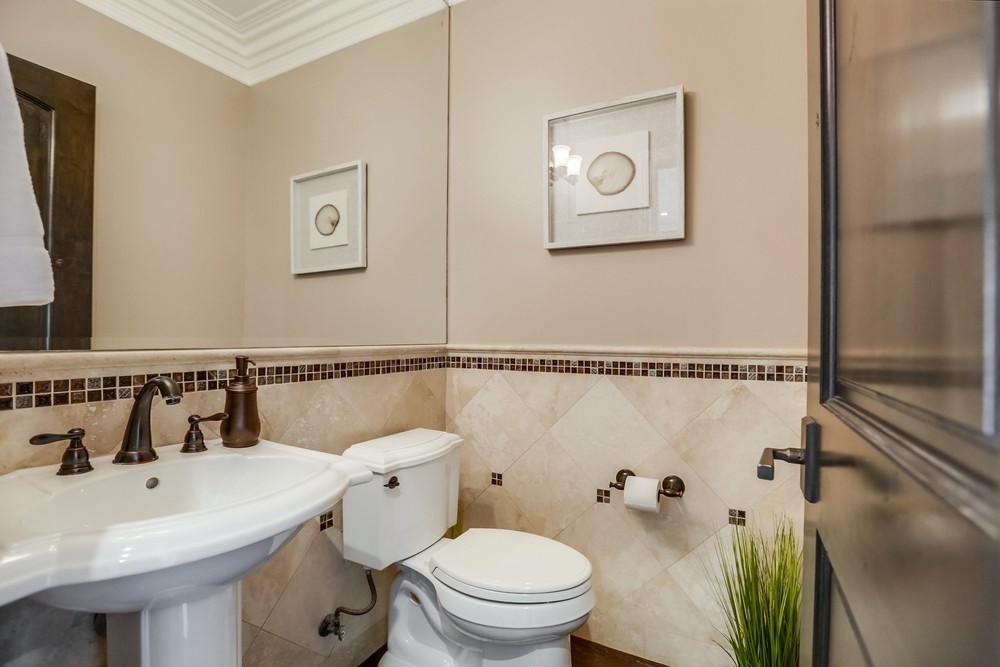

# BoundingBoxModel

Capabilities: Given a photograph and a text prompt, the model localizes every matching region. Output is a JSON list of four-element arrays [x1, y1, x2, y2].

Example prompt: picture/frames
[[290, 159, 367, 273], [541, 85, 686, 248]]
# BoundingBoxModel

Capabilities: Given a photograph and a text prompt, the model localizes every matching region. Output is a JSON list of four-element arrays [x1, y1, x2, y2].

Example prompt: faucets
[[112, 375, 183, 465]]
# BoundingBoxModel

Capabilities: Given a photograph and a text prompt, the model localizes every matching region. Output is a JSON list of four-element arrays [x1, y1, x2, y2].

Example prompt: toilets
[[340, 425, 598, 667]]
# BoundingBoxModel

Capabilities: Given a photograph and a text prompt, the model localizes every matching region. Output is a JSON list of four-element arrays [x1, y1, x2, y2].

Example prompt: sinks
[[7, 436, 375, 614]]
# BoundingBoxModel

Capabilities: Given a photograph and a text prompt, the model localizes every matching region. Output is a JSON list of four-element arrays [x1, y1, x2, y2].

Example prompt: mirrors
[[0, 1, 451, 353]]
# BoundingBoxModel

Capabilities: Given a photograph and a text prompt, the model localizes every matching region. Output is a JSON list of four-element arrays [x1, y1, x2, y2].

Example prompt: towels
[[0, 39, 56, 307]]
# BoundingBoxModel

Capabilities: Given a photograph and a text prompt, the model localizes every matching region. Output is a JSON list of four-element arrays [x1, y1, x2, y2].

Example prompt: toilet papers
[[623, 475, 663, 514]]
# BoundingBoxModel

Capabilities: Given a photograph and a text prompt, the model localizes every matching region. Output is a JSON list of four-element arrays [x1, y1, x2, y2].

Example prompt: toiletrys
[[219, 355, 261, 448]]
